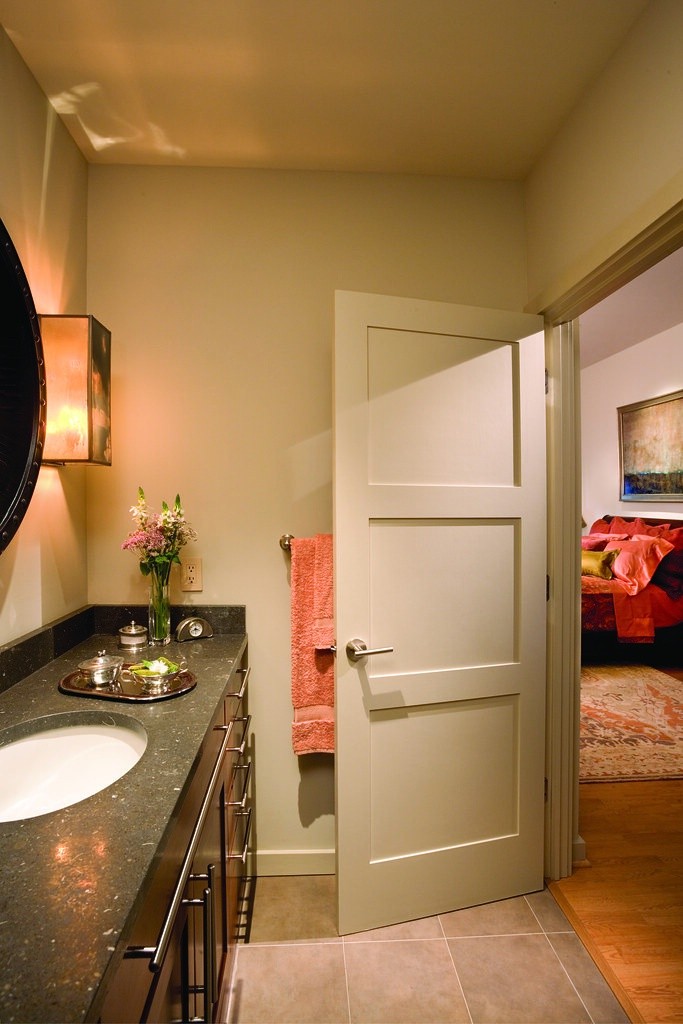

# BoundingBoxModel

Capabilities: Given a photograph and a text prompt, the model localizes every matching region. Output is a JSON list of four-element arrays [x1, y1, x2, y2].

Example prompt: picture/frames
[[616, 390, 683, 507]]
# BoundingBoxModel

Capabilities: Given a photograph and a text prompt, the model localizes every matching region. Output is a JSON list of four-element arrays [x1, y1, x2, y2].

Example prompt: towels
[[289, 532, 333, 757]]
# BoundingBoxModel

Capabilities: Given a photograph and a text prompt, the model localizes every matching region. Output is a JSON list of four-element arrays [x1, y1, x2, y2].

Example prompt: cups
[[78, 650, 124, 685], [121, 661, 189, 687]]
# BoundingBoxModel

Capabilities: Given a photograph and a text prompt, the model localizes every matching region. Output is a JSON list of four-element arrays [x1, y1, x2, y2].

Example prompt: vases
[[144, 559, 172, 648]]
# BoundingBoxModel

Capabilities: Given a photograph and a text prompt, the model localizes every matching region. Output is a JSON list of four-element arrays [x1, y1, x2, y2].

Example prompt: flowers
[[121, 485, 196, 575]]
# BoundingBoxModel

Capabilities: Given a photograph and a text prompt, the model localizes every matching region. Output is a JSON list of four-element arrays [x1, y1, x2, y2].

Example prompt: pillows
[[603, 533, 675, 597], [582, 529, 628, 552]]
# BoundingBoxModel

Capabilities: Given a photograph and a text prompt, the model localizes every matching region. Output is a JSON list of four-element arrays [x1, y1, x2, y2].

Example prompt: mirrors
[[0, 214, 49, 550]]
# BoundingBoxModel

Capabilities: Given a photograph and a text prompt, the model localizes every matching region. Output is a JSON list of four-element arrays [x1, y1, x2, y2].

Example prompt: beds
[[582, 515, 683, 668]]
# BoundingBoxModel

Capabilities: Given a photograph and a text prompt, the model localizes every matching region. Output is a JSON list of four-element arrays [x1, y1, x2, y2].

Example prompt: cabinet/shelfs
[[93, 646, 260, 1024]]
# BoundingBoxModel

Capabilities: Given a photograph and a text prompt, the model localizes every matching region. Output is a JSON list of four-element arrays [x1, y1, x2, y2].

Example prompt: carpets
[[579, 662, 683, 782]]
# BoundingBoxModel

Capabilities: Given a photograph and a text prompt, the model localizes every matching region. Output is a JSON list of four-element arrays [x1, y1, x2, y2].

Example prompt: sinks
[[1, 711, 149, 825]]
